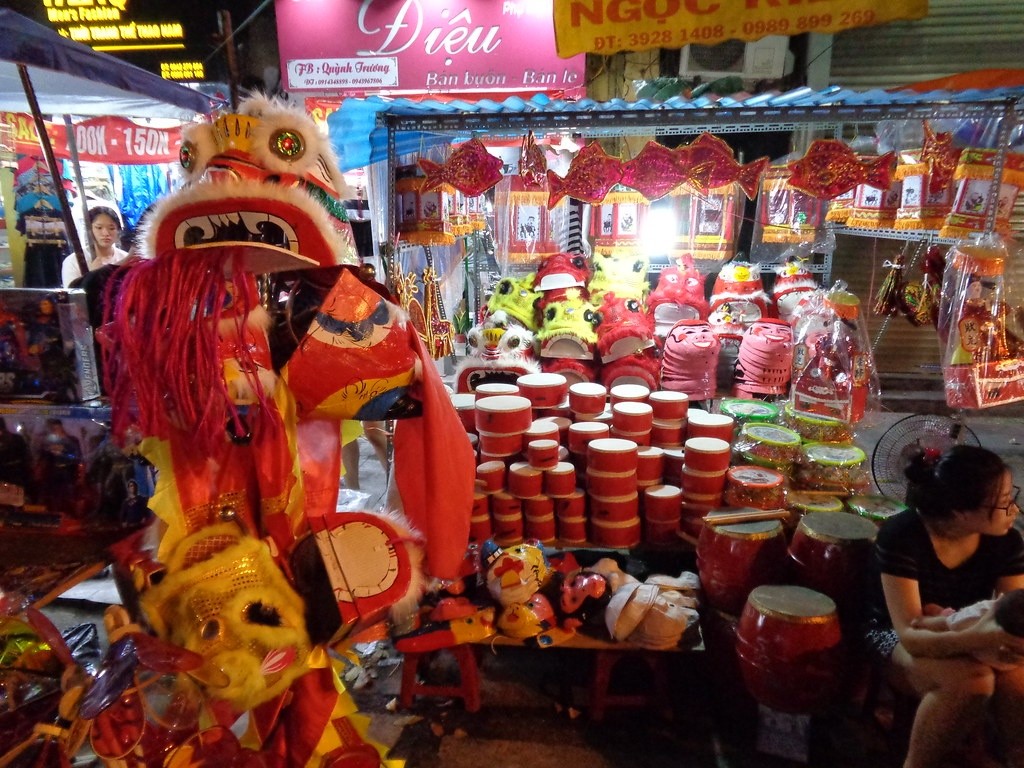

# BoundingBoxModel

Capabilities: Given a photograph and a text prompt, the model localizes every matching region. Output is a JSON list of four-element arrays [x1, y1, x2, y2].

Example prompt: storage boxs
[[0, 287, 100, 404], [0, 397, 157, 520]]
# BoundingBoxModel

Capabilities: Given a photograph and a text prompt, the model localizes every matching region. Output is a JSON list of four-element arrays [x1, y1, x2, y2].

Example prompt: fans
[[871, 413, 981, 500]]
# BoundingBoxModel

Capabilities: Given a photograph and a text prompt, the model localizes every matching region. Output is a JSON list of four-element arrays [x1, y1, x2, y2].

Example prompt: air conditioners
[[679, 34, 790, 81]]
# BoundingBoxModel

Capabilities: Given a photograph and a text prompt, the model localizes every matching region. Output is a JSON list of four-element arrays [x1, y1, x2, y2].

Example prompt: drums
[[687, 390, 916, 721], [445, 369, 740, 551]]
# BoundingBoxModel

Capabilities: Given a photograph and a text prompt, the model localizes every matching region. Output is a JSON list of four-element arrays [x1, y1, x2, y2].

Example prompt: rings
[[1000, 646, 1004, 652]]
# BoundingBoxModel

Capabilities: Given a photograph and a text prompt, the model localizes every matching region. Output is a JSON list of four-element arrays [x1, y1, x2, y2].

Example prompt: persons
[[910, 587, 1024, 672], [856, 446, 1024, 767], [60, 206, 129, 287]]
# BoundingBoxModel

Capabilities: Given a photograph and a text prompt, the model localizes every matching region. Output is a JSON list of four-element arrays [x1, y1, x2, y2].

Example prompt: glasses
[[977, 485, 1021, 516]]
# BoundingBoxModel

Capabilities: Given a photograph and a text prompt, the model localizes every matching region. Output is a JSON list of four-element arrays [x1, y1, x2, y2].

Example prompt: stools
[[400, 642, 481, 712], [592, 651, 679, 724]]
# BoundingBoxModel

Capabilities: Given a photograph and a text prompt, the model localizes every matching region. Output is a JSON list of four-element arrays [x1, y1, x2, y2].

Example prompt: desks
[[417, 549, 706, 719]]
